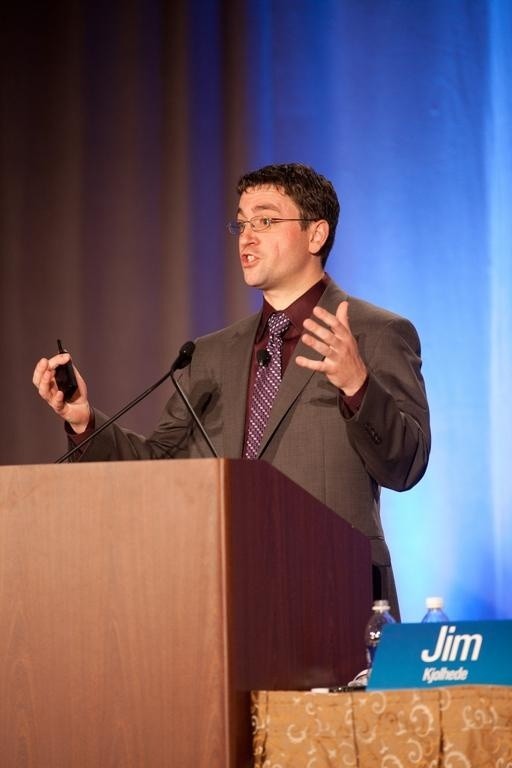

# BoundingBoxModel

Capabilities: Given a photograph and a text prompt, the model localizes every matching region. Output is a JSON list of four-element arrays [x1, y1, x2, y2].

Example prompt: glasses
[[226, 215, 317, 236]]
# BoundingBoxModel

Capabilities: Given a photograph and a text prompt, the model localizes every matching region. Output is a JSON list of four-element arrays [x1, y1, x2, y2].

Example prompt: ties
[[244, 312, 291, 460]]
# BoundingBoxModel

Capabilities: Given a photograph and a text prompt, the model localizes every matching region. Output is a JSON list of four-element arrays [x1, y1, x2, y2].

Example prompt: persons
[[31, 162, 432, 626]]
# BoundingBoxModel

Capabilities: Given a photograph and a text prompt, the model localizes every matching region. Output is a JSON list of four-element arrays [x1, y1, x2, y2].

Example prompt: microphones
[[257, 349, 271, 366], [55, 341, 195, 462], [170, 353, 219, 458]]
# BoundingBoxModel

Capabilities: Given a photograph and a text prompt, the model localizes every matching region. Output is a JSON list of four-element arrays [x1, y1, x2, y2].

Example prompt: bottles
[[363, 599, 394, 664], [423, 593, 447, 622]]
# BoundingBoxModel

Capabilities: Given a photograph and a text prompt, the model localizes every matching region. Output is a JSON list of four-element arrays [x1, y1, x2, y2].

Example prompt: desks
[[248, 685, 511, 767]]
